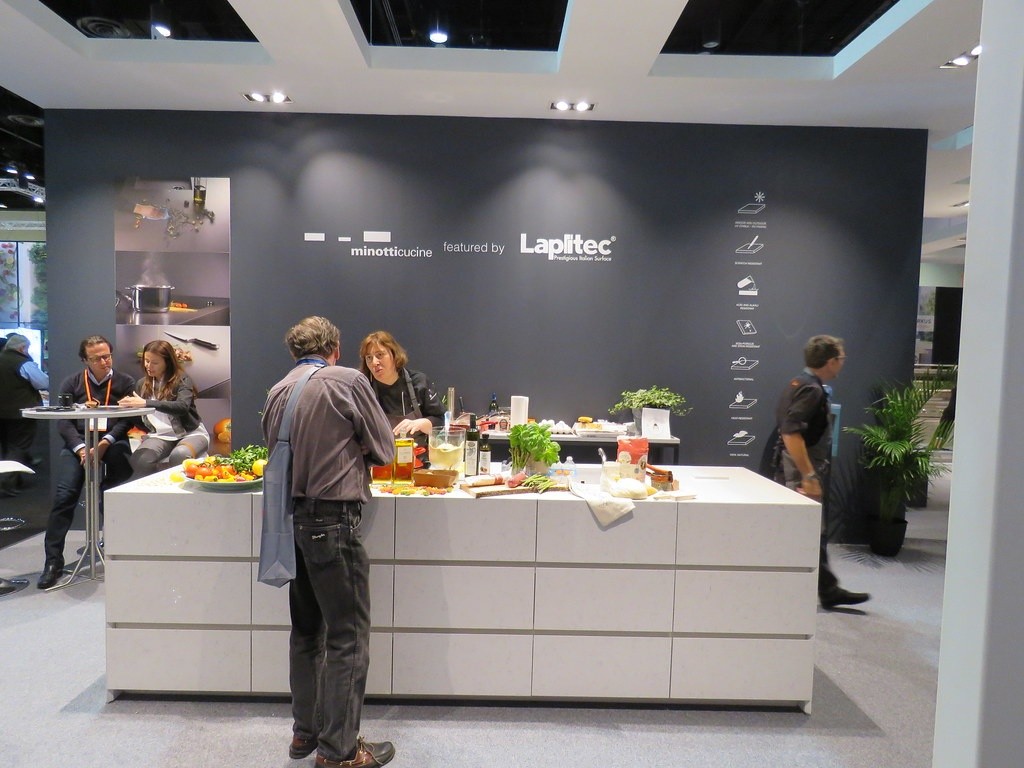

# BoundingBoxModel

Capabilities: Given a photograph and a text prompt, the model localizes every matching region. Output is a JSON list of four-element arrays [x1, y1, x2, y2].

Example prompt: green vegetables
[[214, 444, 269, 472], [507, 420, 561, 475]]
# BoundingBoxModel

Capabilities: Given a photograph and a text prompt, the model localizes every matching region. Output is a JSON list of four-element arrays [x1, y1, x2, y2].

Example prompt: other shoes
[[821, 588, 869, 609], [0, 485, 16, 496]]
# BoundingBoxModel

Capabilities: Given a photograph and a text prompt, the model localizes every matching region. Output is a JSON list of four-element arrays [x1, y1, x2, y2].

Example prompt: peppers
[[170, 462, 254, 483]]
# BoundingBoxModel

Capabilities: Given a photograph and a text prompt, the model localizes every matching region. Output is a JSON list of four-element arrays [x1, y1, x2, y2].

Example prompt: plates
[[181, 470, 263, 490]]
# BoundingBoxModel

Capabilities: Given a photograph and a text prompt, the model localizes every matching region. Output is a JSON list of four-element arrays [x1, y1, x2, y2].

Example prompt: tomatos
[[182, 456, 237, 479], [214, 419, 231, 436], [252, 459, 267, 476]]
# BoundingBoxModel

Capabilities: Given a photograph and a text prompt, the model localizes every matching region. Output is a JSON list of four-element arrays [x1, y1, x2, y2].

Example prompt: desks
[[479, 430, 681, 466], [22, 407, 157, 594]]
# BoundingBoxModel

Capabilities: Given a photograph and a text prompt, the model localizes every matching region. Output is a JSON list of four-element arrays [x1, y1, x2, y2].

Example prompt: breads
[[609, 477, 648, 499]]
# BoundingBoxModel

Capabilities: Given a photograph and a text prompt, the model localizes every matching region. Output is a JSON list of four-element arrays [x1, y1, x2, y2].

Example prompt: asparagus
[[518, 472, 559, 493]]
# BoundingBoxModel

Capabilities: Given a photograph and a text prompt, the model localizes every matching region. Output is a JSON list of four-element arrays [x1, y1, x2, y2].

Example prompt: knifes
[[164, 331, 219, 350]]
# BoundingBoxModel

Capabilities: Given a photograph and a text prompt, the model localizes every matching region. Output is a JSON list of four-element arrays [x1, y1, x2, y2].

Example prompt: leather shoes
[[289, 735, 318, 759], [314, 736, 395, 768], [37, 563, 64, 589]]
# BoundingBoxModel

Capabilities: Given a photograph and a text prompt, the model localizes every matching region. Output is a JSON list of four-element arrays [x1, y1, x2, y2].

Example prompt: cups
[[427, 425, 465, 468], [58, 393, 73, 407], [193, 177, 208, 204]]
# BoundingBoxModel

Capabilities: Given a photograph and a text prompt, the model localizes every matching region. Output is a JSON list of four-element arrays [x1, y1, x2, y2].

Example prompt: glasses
[[86, 353, 113, 363], [835, 355, 848, 362]]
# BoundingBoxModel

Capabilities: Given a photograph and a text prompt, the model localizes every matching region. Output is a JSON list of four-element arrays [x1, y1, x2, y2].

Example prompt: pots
[[126, 285, 175, 313]]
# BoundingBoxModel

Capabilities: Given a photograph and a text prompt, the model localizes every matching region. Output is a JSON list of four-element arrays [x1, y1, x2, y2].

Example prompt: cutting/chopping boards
[[460, 483, 566, 498]]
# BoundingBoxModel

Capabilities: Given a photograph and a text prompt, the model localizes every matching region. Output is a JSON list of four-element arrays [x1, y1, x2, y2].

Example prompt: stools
[[78, 459, 104, 558], [157, 451, 210, 471], [0, 459, 35, 597]]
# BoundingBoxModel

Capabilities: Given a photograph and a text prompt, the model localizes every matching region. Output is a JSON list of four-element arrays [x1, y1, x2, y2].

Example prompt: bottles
[[563, 456, 577, 482], [550, 455, 564, 480], [465, 413, 478, 477], [478, 433, 492, 476], [489, 391, 498, 413], [392, 429, 414, 484], [370, 462, 392, 484]]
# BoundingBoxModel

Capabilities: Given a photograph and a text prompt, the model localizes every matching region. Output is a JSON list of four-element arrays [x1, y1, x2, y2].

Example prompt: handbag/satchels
[[257, 441, 296, 588], [759, 425, 783, 480]]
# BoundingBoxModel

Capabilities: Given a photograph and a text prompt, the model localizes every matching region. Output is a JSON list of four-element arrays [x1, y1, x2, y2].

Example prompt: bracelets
[[802, 472, 818, 482]]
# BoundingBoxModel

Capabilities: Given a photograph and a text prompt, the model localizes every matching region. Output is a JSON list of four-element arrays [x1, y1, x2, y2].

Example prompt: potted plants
[[608, 384, 694, 436], [841, 362, 958, 557], [905, 418, 954, 509]]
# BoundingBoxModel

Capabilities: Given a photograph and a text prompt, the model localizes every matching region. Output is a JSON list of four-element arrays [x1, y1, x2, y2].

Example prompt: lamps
[[21, 167, 36, 181], [6, 162, 19, 175]]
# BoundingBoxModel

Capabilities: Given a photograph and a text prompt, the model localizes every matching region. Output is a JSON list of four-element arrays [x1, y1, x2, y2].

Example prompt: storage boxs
[[412, 469, 459, 488]]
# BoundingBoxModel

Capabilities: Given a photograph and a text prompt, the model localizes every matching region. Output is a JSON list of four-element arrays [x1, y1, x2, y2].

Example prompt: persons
[[358, 331, 446, 466], [38, 335, 137, 588], [117, 341, 211, 484], [0, 332, 50, 497], [777, 335, 870, 609], [261, 316, 397, 768]]
